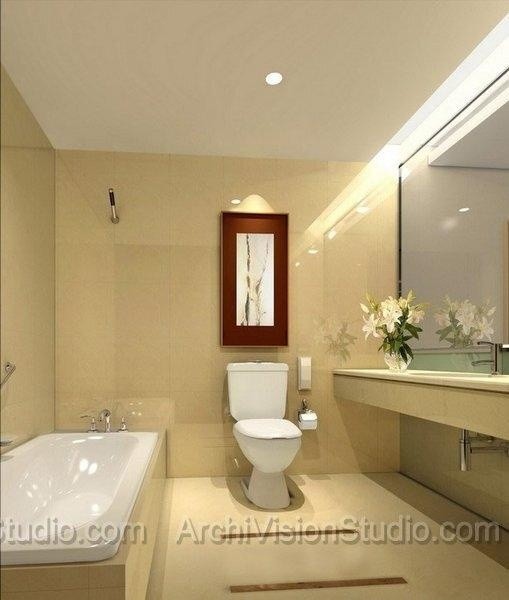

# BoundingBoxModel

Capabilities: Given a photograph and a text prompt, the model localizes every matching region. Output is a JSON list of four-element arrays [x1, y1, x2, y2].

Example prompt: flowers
[[435, 296, 496, 367], [360, 290, 426, 365]]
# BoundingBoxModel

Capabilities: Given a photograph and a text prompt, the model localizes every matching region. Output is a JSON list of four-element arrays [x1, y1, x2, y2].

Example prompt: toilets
[[225, 361, 302, 510]]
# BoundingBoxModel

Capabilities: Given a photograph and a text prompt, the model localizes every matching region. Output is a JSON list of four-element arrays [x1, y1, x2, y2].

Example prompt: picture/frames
[[221, 211, 289, 346]]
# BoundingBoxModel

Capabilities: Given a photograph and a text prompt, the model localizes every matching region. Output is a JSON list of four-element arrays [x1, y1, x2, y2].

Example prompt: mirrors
[[399, 67, 509, 375]]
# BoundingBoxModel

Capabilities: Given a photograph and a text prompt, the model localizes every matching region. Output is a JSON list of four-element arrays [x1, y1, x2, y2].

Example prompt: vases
[[450, 348, 479, 372], [384, 352, 411, 370]]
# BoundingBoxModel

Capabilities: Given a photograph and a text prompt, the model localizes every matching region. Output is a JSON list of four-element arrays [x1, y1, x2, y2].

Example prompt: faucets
[[98, 410, 112, 432], [471, 341, 503, 373]]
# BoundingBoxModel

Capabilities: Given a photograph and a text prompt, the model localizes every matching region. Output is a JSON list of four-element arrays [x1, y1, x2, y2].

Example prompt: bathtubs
[[0, 430, 159, 566]]
